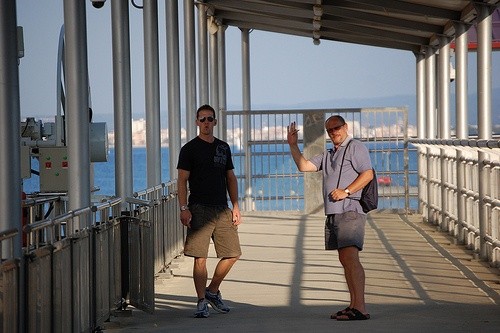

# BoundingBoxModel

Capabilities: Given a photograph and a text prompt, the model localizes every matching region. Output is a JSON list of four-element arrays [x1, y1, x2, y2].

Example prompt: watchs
[[180, 205, 189, 211], [345, 188, 350, 196]]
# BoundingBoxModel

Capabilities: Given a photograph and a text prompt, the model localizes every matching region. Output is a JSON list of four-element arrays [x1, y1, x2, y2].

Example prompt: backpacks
[[336, 139, 378, 213]]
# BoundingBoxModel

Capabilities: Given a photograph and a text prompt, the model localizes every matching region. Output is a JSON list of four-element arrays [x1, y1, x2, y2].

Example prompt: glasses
[[199, 116, 214, 122], [327, 124, 346, 133]]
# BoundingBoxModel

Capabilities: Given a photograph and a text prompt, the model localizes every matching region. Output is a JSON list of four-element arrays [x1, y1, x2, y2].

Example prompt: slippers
[[337, 308, 370, 321], [331, 307, 351, 319]]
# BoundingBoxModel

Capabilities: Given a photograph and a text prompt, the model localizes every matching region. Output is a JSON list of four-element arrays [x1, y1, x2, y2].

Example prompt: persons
[[287, 115, 374, 321], [176, 105, 243, 318]]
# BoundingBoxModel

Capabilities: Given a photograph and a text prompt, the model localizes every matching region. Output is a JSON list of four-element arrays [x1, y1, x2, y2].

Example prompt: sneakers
[[197, 300, 210, 317], [205, 287, 230, 313]]
